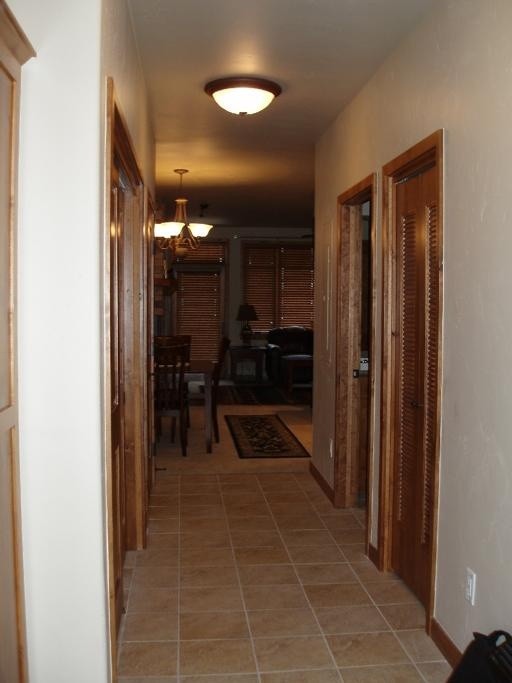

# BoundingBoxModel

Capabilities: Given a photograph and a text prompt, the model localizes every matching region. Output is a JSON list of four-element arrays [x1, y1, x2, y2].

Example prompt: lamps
[[203, 74, 283, 116], [235, 302, 259, 343], [153, 167, 214, 257]]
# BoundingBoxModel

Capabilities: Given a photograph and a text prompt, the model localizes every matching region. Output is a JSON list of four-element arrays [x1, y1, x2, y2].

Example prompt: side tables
[[281, 354, 312, 403]]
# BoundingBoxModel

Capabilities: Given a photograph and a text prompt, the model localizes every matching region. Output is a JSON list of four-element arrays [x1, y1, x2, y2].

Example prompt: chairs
[[267, 325, 314, 400], [152, 332, 233, 457]]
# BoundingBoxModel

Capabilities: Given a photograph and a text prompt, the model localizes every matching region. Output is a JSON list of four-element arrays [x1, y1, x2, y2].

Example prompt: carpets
[[222, 412, 311, 460]]
